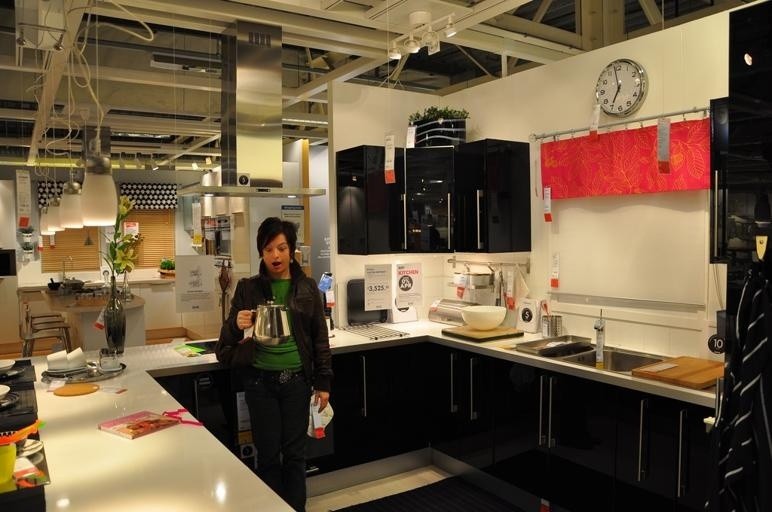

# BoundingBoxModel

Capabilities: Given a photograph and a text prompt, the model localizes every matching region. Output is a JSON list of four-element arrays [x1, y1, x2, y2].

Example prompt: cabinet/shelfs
[[305, 342, 429, 478], [709, 97, 772, 263], [425, 340, 494, 477], [153, 370, 234, 455], [728, 0, 772, 97], [494, 357, 616, 511], [335, 138, 531, 256], [616, 385, 715, 512]]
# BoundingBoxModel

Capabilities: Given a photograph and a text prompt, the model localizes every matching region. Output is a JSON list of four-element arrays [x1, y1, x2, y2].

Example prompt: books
[[99, 411, 180, 439]]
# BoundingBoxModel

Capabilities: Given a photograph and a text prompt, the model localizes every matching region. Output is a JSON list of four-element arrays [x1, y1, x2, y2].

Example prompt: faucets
[[594, 319, 605, 369]]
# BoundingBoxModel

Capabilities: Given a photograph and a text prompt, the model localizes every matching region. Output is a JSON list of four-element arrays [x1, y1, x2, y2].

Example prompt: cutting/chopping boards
[[439, 325, 525, 341], [631, 355, 724, 389]]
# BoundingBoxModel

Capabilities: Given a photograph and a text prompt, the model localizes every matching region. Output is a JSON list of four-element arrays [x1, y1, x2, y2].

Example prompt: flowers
[[97, 195, 145, 347]]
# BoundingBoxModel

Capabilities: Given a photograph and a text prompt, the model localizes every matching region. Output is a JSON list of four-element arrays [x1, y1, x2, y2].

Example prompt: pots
[[66, 276, 92, 289], [48, 277, 61, 291]]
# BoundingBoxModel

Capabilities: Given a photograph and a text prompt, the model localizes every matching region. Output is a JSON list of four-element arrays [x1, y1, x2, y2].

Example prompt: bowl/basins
[[461, 305, 508, 331], [1, 384, 11, 400], [94, 281, 104, 289], [452, 271, 491, 287], [0, 359, 16, 371]]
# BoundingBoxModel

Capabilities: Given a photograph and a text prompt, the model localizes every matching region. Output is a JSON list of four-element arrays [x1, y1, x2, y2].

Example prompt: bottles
[[122, 270, 132, 302]]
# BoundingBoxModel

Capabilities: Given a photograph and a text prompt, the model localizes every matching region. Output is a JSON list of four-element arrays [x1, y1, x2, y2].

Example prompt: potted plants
[[409, 106, 472, 147], [17, 225, 35, 242], [157, 260, 175, 279], [21, 242, 34, 258]]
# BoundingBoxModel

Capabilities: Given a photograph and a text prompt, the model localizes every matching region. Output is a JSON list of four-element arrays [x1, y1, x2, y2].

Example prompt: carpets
[[328, 475, 528, 512]]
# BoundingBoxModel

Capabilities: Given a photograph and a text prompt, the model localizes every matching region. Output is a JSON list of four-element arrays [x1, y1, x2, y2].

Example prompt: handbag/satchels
[[215, 323, 252, 367]]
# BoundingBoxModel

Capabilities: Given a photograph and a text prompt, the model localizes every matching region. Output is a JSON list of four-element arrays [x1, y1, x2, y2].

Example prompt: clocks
[[595, 58, 649, 118]]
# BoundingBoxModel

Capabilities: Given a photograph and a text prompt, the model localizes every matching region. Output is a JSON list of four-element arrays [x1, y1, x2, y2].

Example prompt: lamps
[[390, 10, 458, 60], [39, 0, 118, 236], [66, 140, 221, 171]]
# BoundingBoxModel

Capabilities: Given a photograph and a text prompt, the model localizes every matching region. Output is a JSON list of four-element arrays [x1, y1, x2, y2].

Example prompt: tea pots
[[248, 299, 290, 344]]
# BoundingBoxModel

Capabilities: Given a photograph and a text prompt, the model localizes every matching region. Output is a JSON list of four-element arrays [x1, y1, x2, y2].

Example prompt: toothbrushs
[[542, 301, 549, 315]]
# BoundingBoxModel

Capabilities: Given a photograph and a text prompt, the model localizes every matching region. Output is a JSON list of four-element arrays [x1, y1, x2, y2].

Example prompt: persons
[[215, 215, 333, 512]]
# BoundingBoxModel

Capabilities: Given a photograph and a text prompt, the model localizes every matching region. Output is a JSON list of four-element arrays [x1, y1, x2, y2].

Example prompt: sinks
[[562, 345, 664, 374]]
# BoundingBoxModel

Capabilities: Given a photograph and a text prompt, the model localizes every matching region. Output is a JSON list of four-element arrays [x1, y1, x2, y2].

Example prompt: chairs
[[17, 291, 72, 357]]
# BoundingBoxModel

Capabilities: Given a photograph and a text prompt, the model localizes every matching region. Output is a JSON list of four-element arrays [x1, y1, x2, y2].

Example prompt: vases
[[104, 276, 126, 353]]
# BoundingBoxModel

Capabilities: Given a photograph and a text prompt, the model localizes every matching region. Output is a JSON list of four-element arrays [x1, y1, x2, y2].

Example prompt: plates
[[16, 437, 45, 458], [0, 390, 20, 410], [30, 451, 44, 465], [41, 362, 125, 383], [0, 364, 25, 381]]
